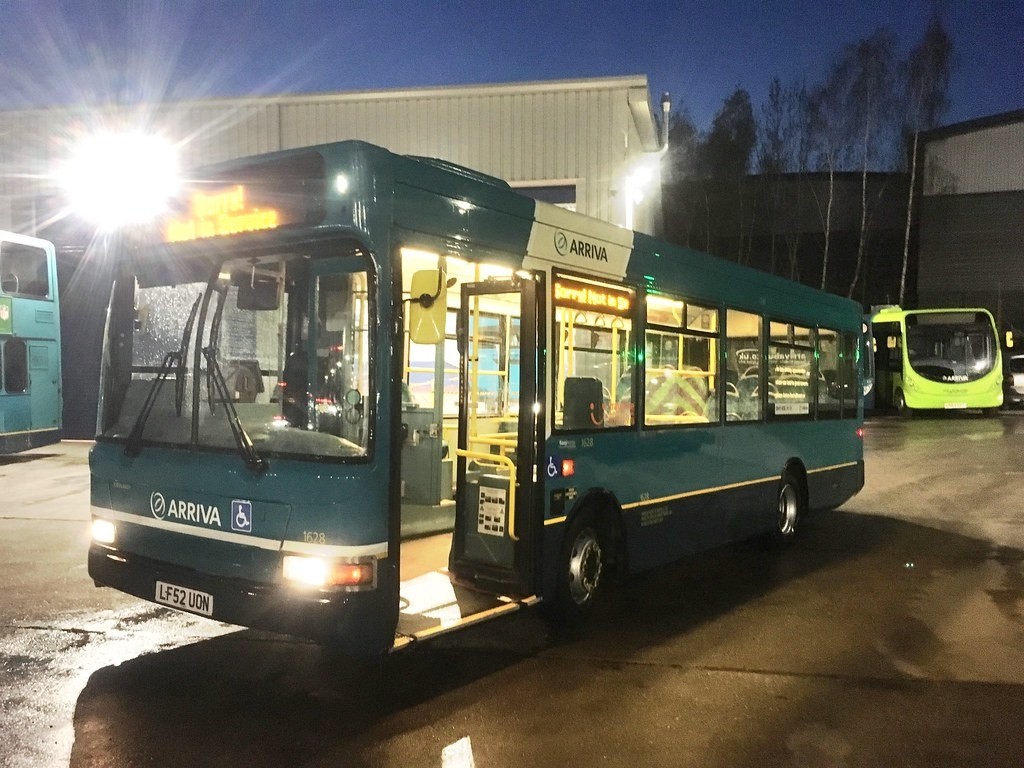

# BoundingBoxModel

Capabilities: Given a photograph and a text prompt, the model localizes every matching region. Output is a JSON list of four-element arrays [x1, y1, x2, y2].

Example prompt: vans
[[1004, 354, 1024, 408]]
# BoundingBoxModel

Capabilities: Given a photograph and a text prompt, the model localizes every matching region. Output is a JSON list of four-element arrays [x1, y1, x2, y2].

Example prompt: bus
[[88, 140, 866, 670], [870, 303, 1014, 418], [1, 231, 61, 454]]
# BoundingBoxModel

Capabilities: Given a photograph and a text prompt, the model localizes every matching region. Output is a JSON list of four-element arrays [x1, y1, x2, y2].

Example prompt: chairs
[[646, 365, 676, 415], [819, 369, 838, 398], [616, 365, 632, 403], [617, 387, 634, 425], [676, 365, 707, 415], [677, 409, 700, 423], [775, 367, 810, 413], [725, 370, 738, 392], [726, 413, 741, 422], [602, 386, 612, 421], [742, 381, 782, 421], [702, 382, 738, 420], [803, 363, 830, 405], [737, 366, 770, 403]]
